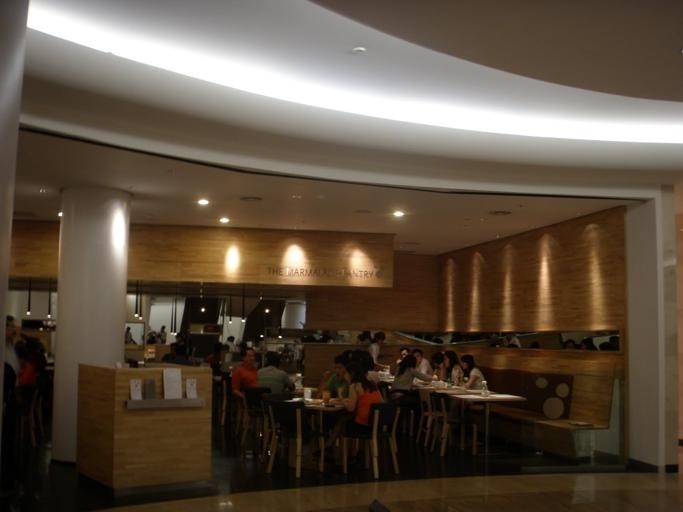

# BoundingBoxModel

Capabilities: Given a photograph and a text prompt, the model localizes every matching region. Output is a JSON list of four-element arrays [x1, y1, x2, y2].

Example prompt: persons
[[206, 326, 384, 463], [2, 315, 48, 498], [489, 332, 541, 349], [125, 326, 194, 367], [565, 336, 618, 352], [368, 331, 488, 432]]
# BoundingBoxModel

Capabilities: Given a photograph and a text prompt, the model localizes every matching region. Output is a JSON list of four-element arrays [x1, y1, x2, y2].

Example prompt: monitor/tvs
[[123, 316, 147, 363]]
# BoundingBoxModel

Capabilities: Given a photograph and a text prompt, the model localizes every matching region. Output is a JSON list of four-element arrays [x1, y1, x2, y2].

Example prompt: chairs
[[214, 379, 477, 478], [18, 389, 38, 449]]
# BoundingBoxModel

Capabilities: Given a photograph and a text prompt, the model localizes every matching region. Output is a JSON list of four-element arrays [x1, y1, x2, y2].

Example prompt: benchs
[[469, 369, 620, 466]]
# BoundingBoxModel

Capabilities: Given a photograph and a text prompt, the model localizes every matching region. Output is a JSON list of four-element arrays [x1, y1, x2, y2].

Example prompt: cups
[[322, 390, 331, 404], [303, 387, 312, 400]]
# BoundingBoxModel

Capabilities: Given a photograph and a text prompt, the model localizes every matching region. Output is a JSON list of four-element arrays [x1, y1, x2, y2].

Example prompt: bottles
[[481, 380, 487, 396]]
